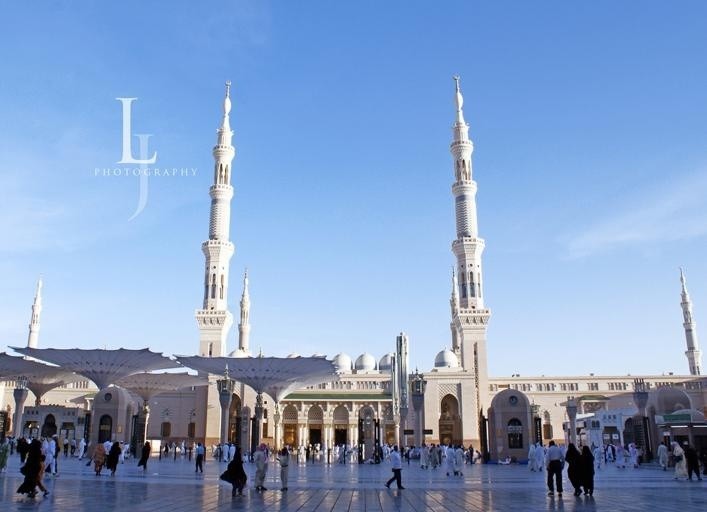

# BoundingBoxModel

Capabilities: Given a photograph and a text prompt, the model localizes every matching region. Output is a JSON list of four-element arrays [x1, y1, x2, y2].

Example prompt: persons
[[210, 437, 291, 498], [382, 442, 482, 490], [527, 439, 644, 498], [0, 432, 205, 504], [656, 440, 702, 483]]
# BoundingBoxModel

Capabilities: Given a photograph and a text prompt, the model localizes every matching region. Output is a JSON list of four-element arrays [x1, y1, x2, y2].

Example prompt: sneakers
[[398, 486, 405, 489], [281, 488, 287, 491], [261, 486, 266, 490], [385, 484, 389, 488], [548, 490, 593, 496], [43, 491, 49, 496]]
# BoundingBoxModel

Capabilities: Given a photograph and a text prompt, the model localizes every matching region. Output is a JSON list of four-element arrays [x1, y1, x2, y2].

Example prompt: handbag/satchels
[[219, 471, 236, 484]]
